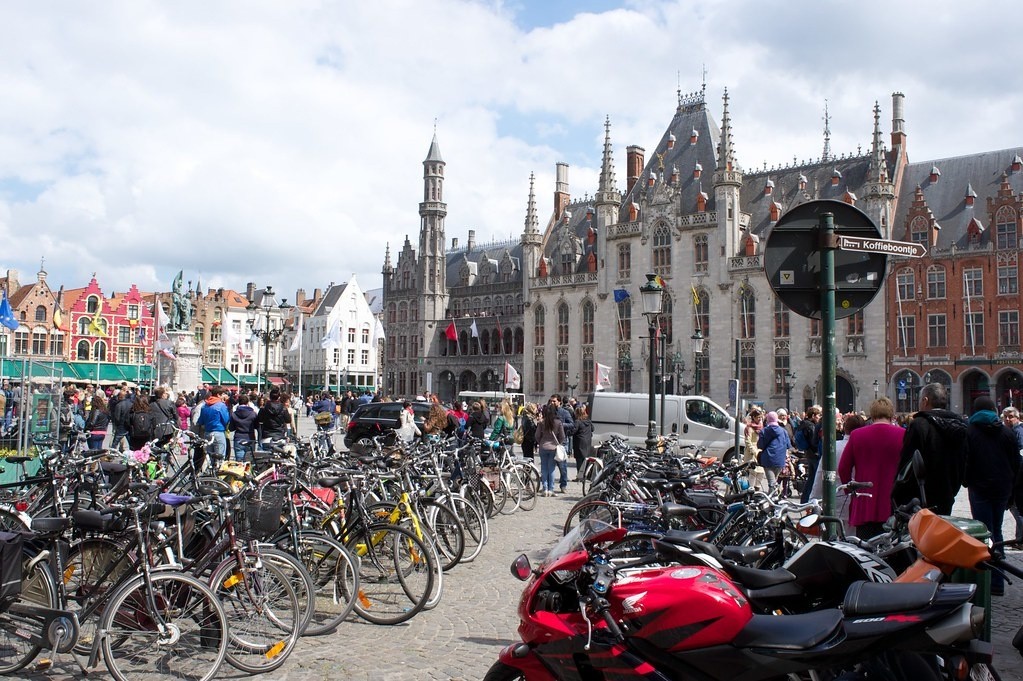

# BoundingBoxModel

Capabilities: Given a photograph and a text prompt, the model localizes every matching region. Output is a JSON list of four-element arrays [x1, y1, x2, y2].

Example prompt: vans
[[585, 393, 747, 467]]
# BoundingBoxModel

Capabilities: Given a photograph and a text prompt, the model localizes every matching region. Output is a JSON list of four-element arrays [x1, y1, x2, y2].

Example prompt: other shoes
[[1012, 544, 1023, 551], [561, 487, 569, 494], [327, 447, 335, 454]]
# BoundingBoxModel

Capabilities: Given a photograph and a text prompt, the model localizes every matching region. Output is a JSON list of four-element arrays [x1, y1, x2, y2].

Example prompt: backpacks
[[393, 414, 405, 429], [794, 427, 808, 450], [513, 423, 526, 444], [132, 411, 152, 433], [454, 413, 466, 432]]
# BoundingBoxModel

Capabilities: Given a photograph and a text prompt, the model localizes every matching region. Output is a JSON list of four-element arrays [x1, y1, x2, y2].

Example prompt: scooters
[[883, 496, 1023, 681]]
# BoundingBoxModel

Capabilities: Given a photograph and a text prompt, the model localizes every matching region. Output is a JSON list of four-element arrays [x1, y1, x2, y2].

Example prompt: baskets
[[235, 488, 284, 541], [481, 441, 503, 467], [451, 467, 480, 491]]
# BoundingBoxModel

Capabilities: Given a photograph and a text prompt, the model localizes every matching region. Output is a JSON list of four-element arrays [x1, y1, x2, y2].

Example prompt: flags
[[497, 319, 504, 340], [470, 319, 480, 338], [154, 300, 172, 351], [505, 362, 521, 390], [320, 316, 343, 350], [372, 318, 387, 347], [595, 363, 613, 392], [288, 318, 304, 351], [655, 273, 667, 290], [220, 313, 240, 345], [0, 290, 20, 331], [52, 301, 72, 334], [613, 289, 630, 302], [445, 323, 458, 341], [249, 317, 264, 343], [691, 286, 700, 306], [88, 298, 107, 337]]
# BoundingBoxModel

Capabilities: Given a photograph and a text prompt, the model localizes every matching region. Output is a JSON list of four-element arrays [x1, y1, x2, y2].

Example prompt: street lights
[[691, 327, 705, 394], [907, 370, 912, 412], [487, 365, 504, 411], [638, 272, 665, 453], [446, 372, 460, 402], [140, 354, 151, 389], [246, 285, 294, 393], [89, 368, 94, 384], [563, 372, 581, 454], [671, 352, 697, 396], [872, 379, 880, 399]]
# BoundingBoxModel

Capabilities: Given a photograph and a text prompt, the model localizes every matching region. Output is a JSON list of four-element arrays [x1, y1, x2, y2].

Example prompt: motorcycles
[[484, 519, 1000, 681]]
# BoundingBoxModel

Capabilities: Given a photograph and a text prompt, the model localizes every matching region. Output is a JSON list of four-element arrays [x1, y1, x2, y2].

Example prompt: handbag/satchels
[[495, 417, 509, 445], [554, 444, 568, 462], [169, 416, 179, 429], [292, 487, 334, 507], [315, 411, 332, 425], [757, 452, 763, 465], [0, 531, 23, 597]]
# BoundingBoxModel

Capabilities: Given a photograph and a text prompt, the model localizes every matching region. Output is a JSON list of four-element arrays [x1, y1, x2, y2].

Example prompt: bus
[[458, 389, 525, 413]]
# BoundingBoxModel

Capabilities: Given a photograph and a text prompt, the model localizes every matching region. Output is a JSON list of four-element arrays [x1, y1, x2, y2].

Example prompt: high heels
[[572, 477, 583, 482], [542, 490, 558, 497]]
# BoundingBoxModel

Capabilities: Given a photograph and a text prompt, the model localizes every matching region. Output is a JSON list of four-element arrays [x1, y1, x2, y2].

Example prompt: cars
[[343, 401, 450, 448]]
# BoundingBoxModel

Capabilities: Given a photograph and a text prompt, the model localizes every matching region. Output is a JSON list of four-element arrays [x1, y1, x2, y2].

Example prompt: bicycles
[[0, 430, 1023, 681]]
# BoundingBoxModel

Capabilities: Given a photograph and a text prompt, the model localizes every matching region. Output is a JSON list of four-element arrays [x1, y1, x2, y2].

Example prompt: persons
[[169, 274, 190, 331], [0, 375, 595, 498], [688, 382, 1023, 660]]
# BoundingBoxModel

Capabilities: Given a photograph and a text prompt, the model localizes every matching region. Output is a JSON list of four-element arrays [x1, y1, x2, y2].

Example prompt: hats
[[86, 384, 94, 388], [974, 396, 995, 410], [777, 409, 787, 416], [122, 382, 127, 386], [766, 411, 778, 422]]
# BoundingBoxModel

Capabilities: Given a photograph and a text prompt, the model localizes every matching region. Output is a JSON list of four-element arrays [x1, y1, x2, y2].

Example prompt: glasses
[[408, 404, 412, 406]]
